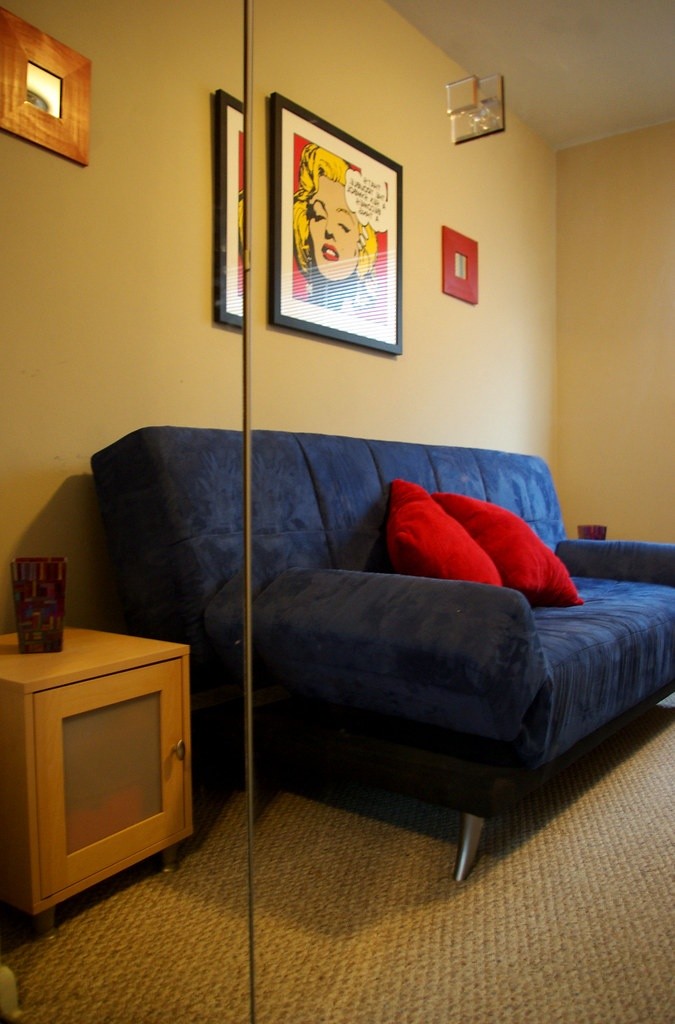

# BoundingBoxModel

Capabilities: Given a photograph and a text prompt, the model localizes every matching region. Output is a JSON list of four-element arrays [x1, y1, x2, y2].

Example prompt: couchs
[[93, 426, 675, 883]]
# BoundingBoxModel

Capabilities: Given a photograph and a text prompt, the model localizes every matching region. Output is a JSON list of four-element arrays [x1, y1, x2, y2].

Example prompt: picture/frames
[[0, 7, 92, 167], [268, 92, 404, 356], [442, 225, 479, 304], [214, 89, 245, 329]]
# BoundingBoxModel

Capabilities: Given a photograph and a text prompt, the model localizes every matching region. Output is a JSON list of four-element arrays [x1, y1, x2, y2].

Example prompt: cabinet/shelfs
[[0, 628, 194, 935]]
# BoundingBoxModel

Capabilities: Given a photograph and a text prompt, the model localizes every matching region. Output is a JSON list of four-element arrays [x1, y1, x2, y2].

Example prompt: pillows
[[429, 491, 583, 609], [385, 479, 502, 586]]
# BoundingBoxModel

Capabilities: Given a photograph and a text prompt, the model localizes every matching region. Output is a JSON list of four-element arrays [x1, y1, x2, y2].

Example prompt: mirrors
[[0, 0, 674, 1024]]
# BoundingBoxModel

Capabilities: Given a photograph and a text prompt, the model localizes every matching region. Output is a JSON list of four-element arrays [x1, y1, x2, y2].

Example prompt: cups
[[577, 525, 607, 540], [10, 556, 66, 654]]
